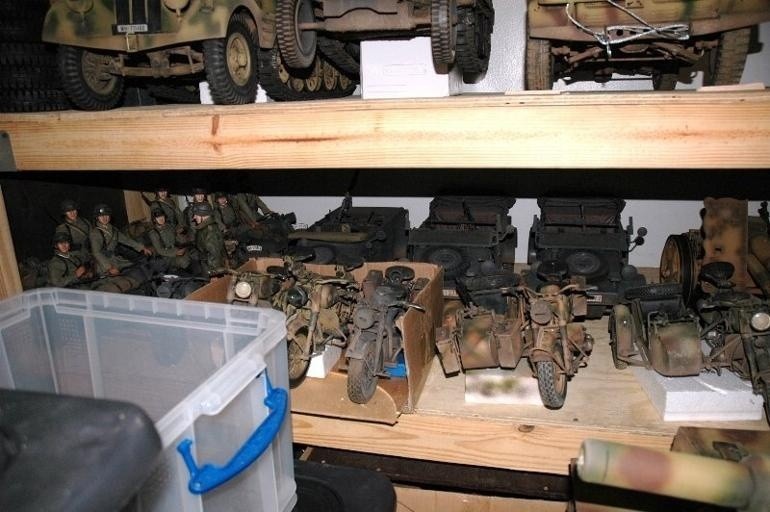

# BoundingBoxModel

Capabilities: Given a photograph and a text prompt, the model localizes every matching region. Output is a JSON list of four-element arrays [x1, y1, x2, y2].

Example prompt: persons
[[47, 179, 279, 299]]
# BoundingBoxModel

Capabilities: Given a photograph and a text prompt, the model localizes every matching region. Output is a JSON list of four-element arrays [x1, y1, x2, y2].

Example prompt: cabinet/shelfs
[[1, 0, 767, 511]]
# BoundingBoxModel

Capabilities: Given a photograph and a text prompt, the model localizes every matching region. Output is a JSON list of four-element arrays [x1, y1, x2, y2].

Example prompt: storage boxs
[[0, 289, 299, 511]]
